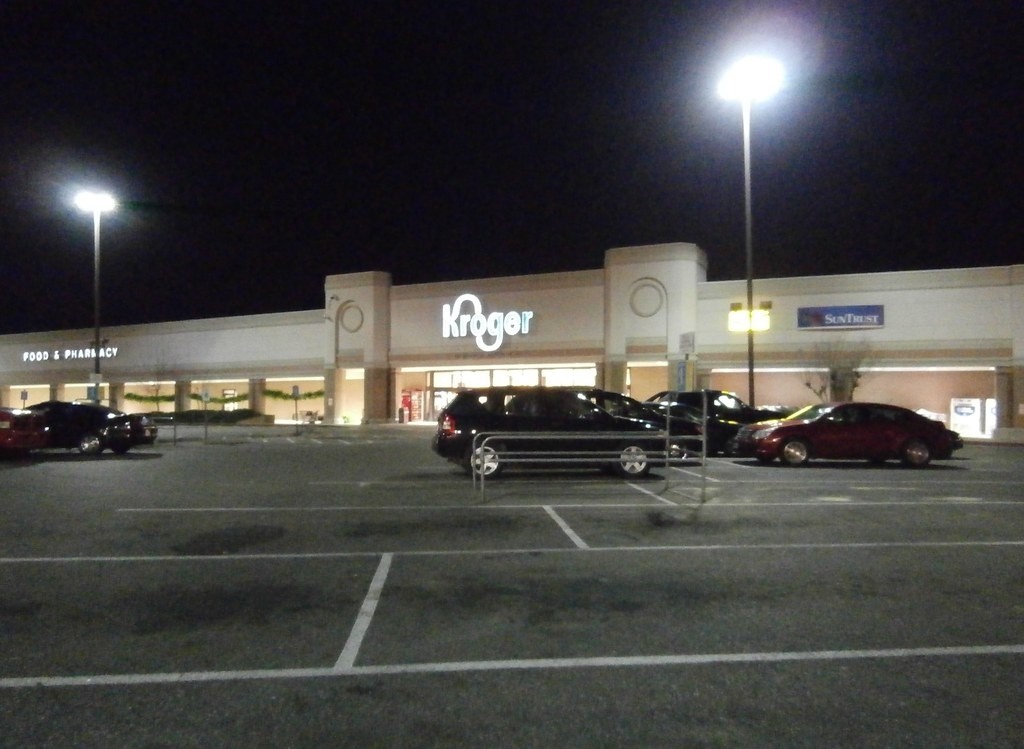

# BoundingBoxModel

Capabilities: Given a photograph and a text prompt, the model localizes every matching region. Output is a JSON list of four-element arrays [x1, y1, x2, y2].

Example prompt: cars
[[0, 406, 48, 460], [733, 400, 953, 469], [27, 400, 159, 457]]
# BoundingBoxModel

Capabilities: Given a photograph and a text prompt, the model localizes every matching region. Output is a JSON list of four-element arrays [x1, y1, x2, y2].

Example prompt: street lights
[[715, 49, 789, 408], [74, 188, 115, 407]]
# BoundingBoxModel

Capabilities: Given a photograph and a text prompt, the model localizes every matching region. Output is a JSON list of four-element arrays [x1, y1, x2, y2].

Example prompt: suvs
[[432, 385, 799, 482]]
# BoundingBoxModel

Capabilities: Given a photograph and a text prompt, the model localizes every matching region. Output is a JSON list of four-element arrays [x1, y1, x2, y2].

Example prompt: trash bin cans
[[399, 406, 410, 423]]
